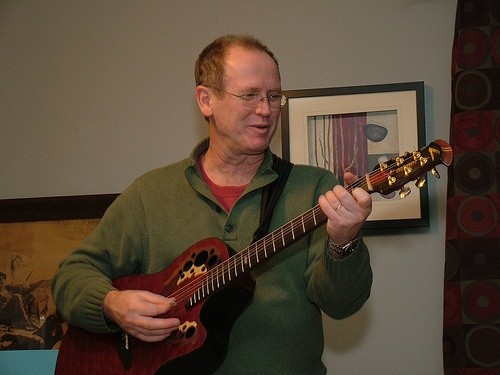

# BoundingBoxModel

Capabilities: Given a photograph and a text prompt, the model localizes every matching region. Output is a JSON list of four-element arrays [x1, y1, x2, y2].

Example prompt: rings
[[335, 204, 342, 211]]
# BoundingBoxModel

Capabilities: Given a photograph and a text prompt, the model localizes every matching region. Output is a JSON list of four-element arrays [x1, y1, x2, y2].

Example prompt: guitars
[[54, 138, 454, 375]]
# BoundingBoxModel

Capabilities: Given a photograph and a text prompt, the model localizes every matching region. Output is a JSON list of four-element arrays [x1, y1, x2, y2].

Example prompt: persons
[[51, 34, 373, 375]]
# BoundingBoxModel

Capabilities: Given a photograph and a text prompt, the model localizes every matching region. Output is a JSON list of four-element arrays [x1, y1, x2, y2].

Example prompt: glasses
[[200, 84, 288, 109]]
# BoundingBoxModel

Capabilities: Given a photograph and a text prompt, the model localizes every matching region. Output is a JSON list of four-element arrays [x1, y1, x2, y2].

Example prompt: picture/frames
[[0, 191, 126, 353], [279, 80, 431, 235]]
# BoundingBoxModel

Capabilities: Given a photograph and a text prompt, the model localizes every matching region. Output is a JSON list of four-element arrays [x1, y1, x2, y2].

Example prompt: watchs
[[328, 236, 359, 255]]
